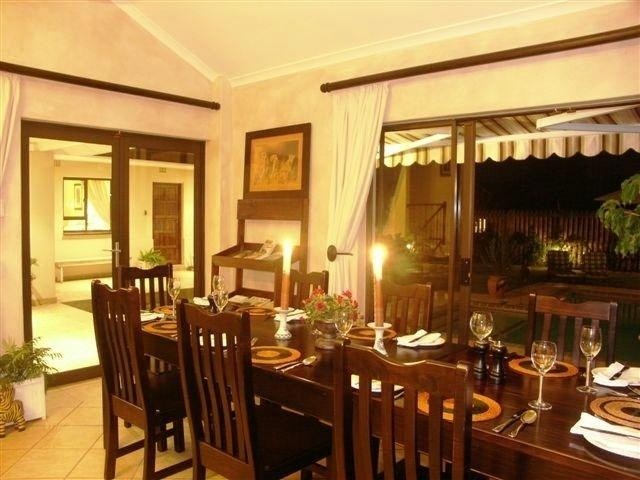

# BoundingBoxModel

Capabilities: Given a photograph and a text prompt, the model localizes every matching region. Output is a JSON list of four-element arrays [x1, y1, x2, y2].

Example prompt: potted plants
[[0, 335, 64, 427], [138, 247, 167, 271], [477, 239, 519, 299]]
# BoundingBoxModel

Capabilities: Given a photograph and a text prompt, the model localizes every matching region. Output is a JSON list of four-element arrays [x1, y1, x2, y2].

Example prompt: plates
[[199, 332, 240, 347], [395, 334, 445, 347], [585, 424, 640, 463], [138, 311, 158, 325], [350, 372, 405, 392], [590, 366, 639, 388]]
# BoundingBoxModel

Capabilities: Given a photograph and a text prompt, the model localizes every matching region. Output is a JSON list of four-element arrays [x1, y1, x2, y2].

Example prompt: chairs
[[546, 250, 610, 287]]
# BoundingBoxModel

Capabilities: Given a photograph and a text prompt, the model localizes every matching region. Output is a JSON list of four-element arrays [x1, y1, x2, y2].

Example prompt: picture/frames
[[242, 122, 312, 199]]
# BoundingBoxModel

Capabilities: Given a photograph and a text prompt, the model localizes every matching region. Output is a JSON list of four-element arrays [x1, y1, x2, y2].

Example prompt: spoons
[[509, 409, 538, 440], [282, 355, 317, 374]]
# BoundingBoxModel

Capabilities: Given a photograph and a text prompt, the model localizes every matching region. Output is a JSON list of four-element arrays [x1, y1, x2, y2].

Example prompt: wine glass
[[575, 323, 602, 396], [527, 339, 558, 411], [167, 274, 229, 319], [468, 311, 494, 341]]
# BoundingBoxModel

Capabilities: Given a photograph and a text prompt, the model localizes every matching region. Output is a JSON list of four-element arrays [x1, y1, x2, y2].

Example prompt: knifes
[[407, 330, 433, 344], [605, 358, 638, 380], [579, 424, 640, 442]]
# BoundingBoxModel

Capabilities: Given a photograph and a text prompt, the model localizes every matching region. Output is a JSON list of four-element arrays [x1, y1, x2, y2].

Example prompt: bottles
[[471, 338, 507, 386]]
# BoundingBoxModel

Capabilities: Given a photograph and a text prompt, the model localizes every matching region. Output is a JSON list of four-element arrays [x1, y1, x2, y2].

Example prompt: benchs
[[55, 259, 112, 283]]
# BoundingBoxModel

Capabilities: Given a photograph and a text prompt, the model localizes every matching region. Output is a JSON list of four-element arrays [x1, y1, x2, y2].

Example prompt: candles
[[368, 241, 390, 327], [280, 236, 294, 309]]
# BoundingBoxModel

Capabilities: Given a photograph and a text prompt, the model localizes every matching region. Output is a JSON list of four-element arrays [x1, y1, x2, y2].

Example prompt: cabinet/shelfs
[[211, 200, 309, 309]]
